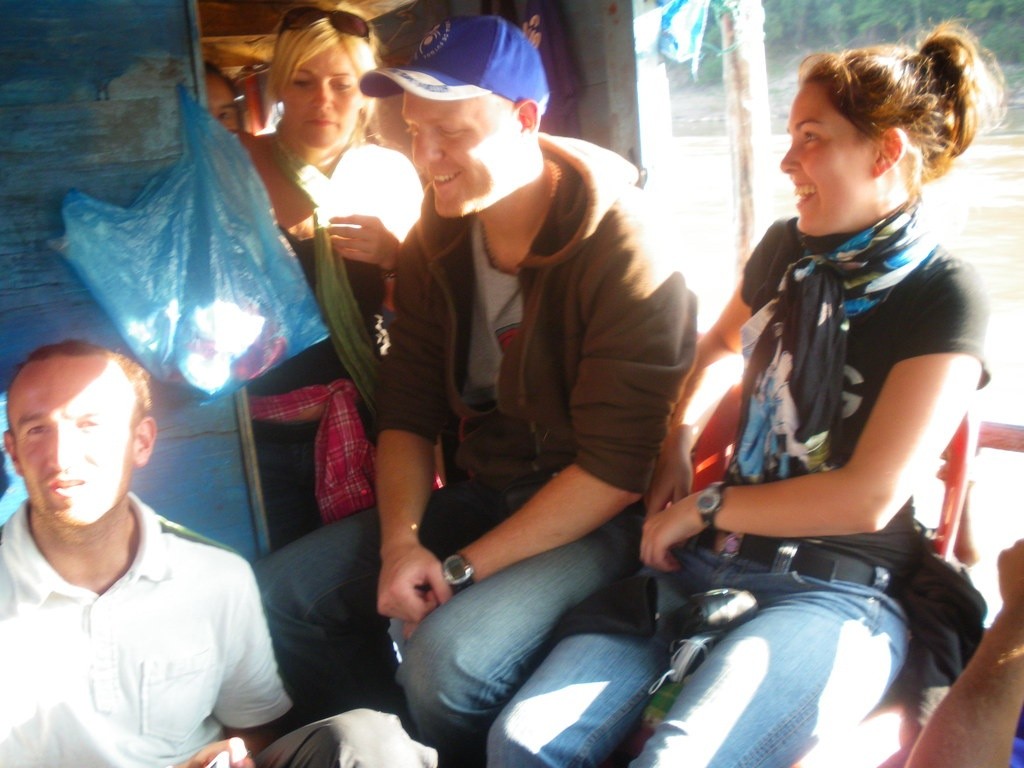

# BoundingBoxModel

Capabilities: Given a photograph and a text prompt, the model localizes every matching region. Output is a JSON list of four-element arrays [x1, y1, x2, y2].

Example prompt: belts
[[692, 527, 904, 598]]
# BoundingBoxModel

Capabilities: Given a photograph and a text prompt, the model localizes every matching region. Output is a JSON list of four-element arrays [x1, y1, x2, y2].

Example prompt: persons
[[1, 339, 437, 768], [902, 538, 1024, 768], [246, 17, 701, 768], [485, 16, 994, 768], [207, 1, 426, 550]]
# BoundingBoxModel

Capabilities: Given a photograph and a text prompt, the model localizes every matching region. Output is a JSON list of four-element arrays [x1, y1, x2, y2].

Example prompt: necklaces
[[482, 159, 560, 273]]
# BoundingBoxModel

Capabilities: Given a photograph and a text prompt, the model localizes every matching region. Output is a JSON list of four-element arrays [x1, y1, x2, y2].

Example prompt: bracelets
[[247, 748, 253, 759]]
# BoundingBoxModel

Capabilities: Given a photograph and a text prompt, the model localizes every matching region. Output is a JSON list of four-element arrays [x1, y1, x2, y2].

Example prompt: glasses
[[276, 8, 370, 50]]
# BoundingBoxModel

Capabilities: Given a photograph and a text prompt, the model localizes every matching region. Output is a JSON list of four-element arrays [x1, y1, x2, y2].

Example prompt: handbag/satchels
[[48, 79, 331, 408]]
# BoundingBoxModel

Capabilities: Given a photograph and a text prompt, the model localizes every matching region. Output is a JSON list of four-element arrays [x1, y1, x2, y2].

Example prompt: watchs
[[697, 481, 726, 529], [443, 552, 474, 594]]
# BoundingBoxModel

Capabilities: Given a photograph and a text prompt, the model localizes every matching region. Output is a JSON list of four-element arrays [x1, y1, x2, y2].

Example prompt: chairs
[[683, 378, 983, 768]]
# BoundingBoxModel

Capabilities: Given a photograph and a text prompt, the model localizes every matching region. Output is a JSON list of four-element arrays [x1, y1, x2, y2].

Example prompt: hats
[[361, 14, 550, 116]]
